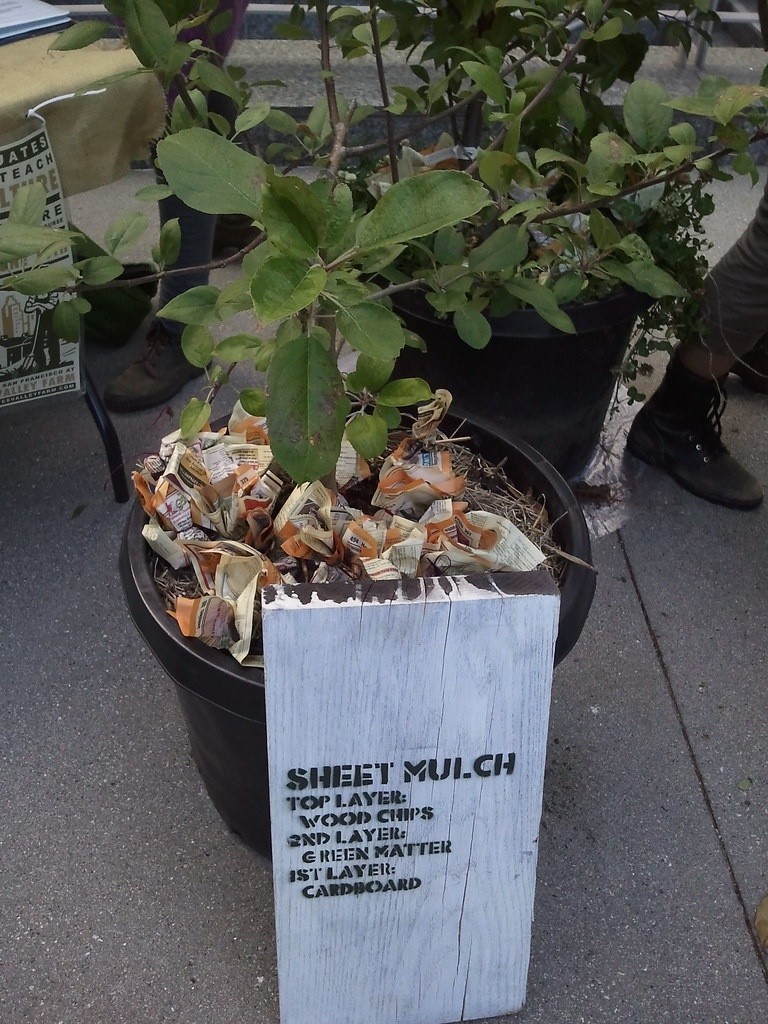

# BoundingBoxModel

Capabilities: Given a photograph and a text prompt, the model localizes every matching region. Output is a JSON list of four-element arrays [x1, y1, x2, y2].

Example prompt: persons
[[104, 0, 252, 411], [628, 186, 768, 510]]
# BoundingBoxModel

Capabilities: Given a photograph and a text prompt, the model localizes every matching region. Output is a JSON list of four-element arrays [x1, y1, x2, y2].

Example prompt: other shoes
[[213, 215, 256, 256], [102, 318, 211, 415]]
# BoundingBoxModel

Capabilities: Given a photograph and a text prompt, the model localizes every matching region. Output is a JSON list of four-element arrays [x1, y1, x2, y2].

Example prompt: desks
[[0, 1, 169, 503]]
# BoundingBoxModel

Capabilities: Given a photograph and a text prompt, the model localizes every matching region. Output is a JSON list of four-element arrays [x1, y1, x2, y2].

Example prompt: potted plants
[[8, 0, 768, 865]]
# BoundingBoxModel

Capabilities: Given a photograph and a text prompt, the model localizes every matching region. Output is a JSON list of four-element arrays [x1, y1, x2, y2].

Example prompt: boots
[[630, 355, 765, 510], [729, 333, 768, 398]]
